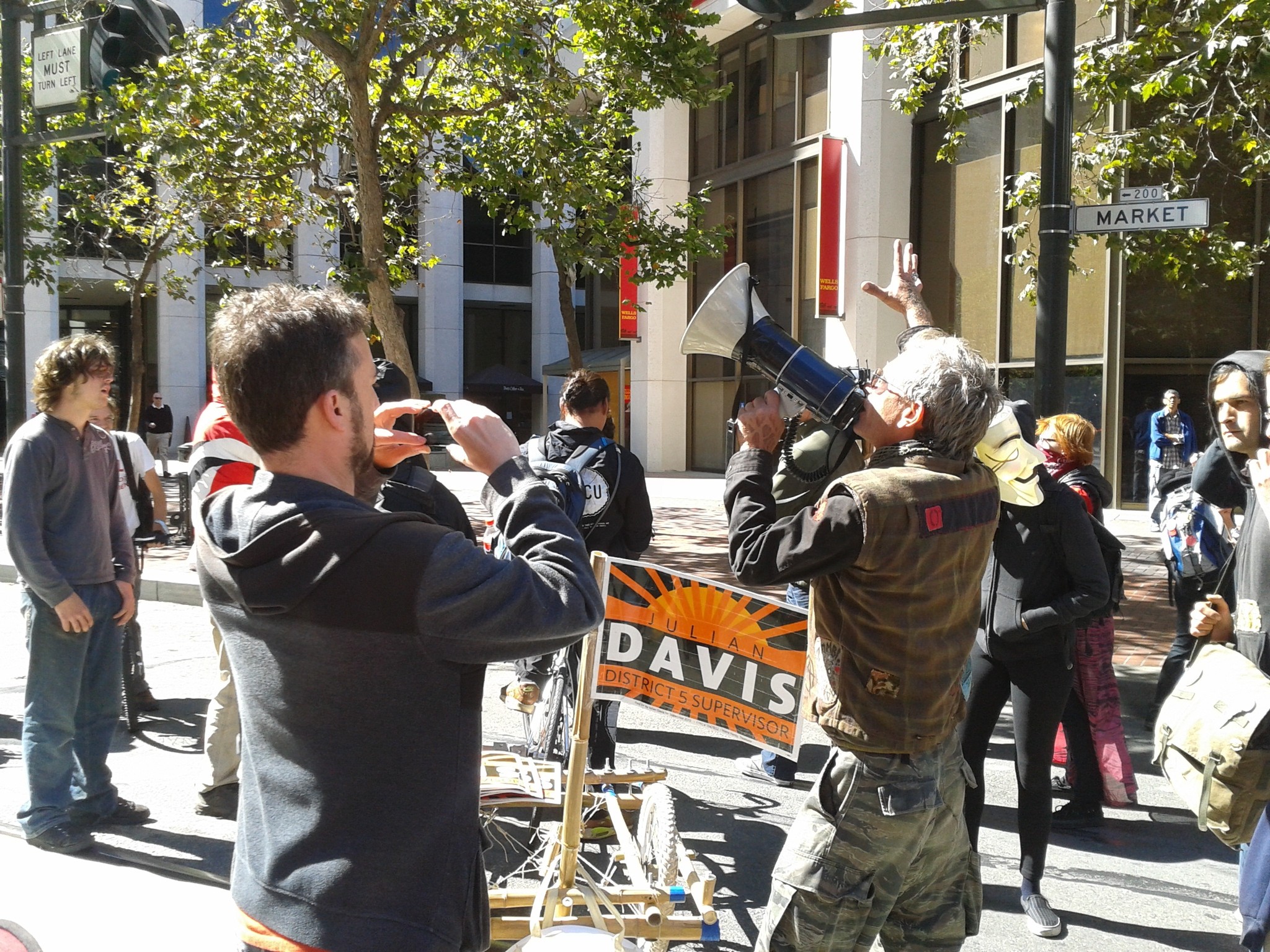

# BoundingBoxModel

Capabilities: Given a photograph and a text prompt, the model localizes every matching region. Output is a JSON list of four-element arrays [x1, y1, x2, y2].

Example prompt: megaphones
[[680, 262, 868, 434]]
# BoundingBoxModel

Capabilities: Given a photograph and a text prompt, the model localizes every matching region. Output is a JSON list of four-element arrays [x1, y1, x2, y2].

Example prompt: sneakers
[[580, 814, 634, 840], [500, 679, 539, 713], [70, 796, 150, 826], [26, 819, 94, 854], [734, 754, 795, 787]]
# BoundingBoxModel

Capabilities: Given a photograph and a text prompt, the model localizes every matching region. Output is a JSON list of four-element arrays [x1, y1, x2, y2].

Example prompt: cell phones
[[411, 408, 460, 448]]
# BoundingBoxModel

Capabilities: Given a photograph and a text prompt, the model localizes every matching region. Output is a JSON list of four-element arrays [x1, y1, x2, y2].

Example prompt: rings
[[912, 272, 918, 280]]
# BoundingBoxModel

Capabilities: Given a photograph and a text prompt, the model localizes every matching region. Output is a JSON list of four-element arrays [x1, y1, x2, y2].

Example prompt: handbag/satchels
[[1150, 640, 1270, 852], [115, 430, 154, 545]]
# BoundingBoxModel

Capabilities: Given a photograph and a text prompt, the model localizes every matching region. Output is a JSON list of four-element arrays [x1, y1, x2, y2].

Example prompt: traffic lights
[[88, 0, 187, 104]]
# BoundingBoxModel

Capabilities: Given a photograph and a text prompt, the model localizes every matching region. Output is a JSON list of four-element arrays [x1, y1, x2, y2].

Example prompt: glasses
[[154, 397, 162, 400], [870, 368, 913, 403], [1039, 433, 1059, 445]]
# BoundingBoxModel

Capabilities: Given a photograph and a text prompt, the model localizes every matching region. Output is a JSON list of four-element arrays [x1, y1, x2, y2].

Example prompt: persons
[[1149, 390, 1195, 532], [1031, 413, 1138, 807], [143, 392, 174, 477], [602, 408, 615, 439], [190, 284, 605, 952], [1130, 397, 1159, 502], [724, 239, 999, 952], [87, 397, 166, 709], [182, 376, 262, 821], [5, 333, 151, 850], [370, 356, 478, 546], [956, 399, 1111, 937], [520, 369, 653, 762], [1189, 356, 1270, 952]]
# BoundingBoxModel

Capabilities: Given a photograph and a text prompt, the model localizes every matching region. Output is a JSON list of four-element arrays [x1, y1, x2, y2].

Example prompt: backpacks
[[493, 433, 616, 563], [1158, 481, 1231, 579]]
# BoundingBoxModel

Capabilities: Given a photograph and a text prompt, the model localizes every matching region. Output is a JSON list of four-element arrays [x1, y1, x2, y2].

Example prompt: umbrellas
[[464, 363, 548, 414], [416, 376, 433, 391]]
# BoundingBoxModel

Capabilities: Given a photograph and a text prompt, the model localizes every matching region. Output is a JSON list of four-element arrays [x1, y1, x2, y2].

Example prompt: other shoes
[[1020, 893, 1062, 937], [1149, 521, 1161, 532], [135, 688, 161, 710], [1052, 775, 1072, 794], [1052, 804, 1107, 829], [163, 471, 171, 477], [195, 782, 239, 821]]
